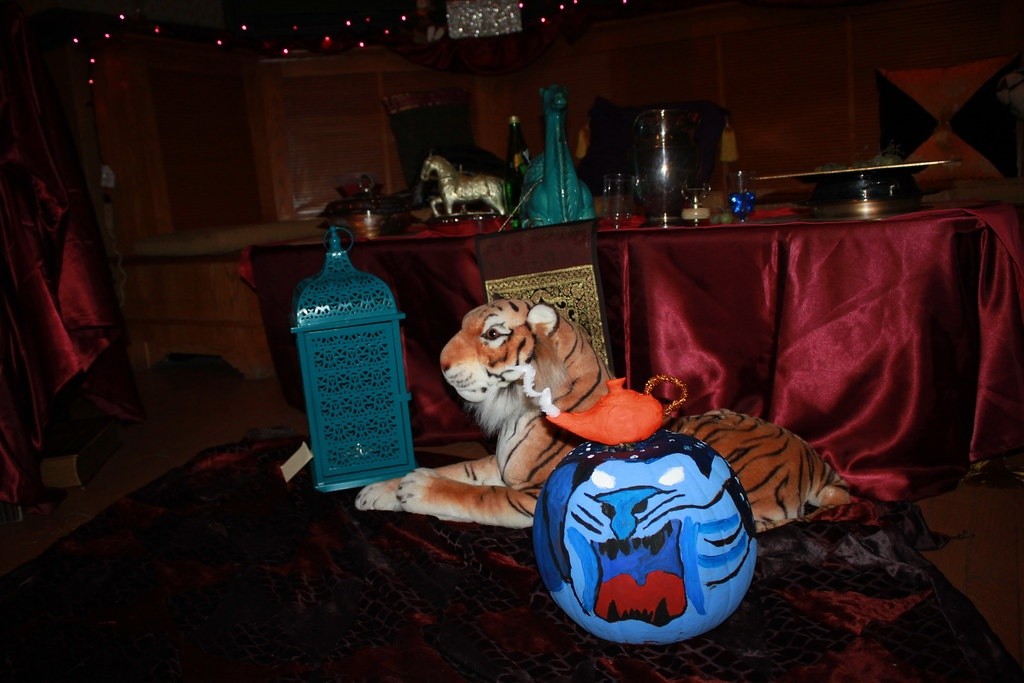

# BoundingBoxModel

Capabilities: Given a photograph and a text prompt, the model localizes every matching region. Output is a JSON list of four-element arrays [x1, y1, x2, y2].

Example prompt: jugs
[[629, 104, 725, 228]]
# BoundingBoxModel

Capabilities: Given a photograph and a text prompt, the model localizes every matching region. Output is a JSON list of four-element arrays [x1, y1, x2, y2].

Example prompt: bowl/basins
[[324, 213, 395, 238]]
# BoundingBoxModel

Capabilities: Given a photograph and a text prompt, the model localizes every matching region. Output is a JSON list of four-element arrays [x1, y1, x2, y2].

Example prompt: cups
[[602, 172, 637, 231], [681, 182, 711, 227], [727, 169, 757, 225]]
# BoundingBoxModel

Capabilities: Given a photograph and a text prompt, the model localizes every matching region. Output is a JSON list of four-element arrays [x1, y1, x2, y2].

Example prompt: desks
[[249, 202, 1023, 468]]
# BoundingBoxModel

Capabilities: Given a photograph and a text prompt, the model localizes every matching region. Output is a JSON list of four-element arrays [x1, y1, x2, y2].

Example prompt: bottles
[[504, 115, 532, 230]]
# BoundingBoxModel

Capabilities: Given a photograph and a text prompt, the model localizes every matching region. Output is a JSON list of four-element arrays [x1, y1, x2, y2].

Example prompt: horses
[[420, 150, 506, 218]]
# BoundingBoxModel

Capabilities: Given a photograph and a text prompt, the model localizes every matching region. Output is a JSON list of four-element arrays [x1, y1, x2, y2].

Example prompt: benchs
[[48, 0, 1024, 381]]
